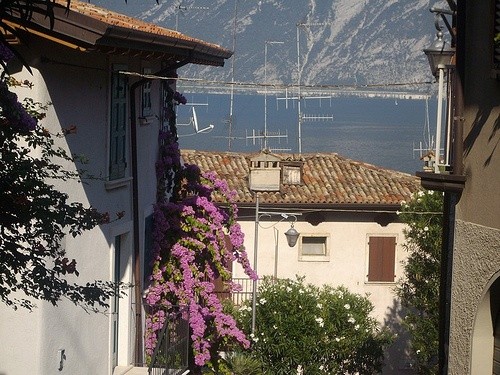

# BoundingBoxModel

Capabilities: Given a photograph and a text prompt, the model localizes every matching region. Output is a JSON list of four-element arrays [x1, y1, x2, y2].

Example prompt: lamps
[[258, 213, 301, 249], [424, 29, 456, 81]]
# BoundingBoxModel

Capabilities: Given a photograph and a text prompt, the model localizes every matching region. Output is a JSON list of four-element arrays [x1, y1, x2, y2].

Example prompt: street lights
[[424, 9, 458, 83], [250, 211, 302, 338]]
